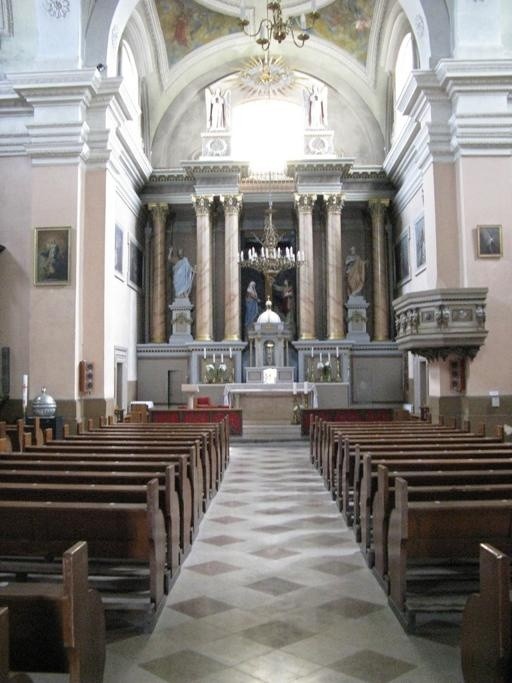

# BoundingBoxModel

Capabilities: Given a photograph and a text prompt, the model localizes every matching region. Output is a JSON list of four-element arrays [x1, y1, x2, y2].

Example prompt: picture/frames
[[34, 226, 71, 286], [126, 232, 145, 294], [114, 221, 127, 281], [393, 225, 412, 289], [413, 209, 427, 275], [477, 224, 503, 258]]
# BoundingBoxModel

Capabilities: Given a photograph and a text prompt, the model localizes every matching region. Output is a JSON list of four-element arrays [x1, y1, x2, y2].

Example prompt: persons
[[168, 247, 197, 301], [46, 238, 64, 259], [344, 247, 368, 296], [244, 280, 261, 327], [283, 279, 295, 325]]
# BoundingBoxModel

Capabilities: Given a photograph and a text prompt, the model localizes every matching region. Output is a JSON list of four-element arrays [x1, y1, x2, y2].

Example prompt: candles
[[238, 245, 304, 260], [311, 346, 339, 362], [203, 347, 233, 363]]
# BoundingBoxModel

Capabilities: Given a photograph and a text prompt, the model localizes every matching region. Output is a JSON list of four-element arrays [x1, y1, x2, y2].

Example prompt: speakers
[[80, 360, 96, 392], [449, 360, 465, 392]]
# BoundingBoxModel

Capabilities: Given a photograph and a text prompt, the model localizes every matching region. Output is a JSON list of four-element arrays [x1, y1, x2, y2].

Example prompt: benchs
[[0, 410, 230, 683], [310, 413, 512, 683]]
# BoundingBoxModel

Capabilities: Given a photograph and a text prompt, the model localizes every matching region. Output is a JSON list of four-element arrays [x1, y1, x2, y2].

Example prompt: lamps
[[238, 1, 321, 49]]
